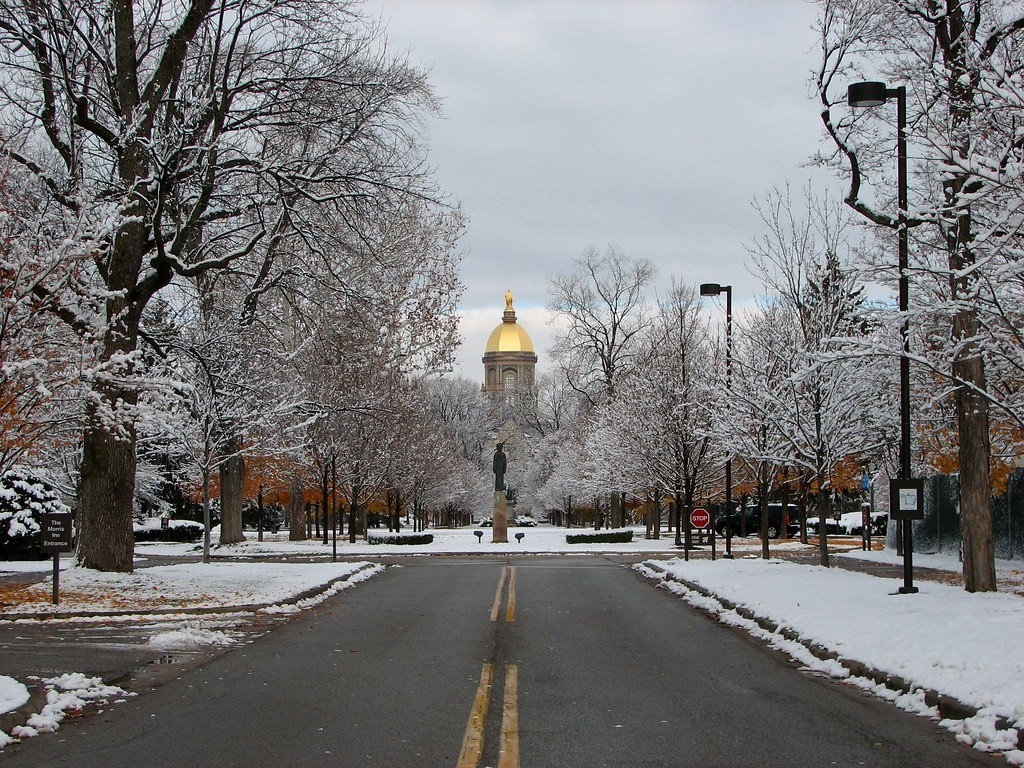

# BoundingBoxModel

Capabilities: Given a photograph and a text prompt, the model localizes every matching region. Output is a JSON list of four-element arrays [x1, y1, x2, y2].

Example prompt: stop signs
[[690, 509, 710, 529]]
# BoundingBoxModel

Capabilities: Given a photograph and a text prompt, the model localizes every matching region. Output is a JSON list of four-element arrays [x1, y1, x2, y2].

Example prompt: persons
[[507, 484, 512, 500], [492, 443, 507, 491]]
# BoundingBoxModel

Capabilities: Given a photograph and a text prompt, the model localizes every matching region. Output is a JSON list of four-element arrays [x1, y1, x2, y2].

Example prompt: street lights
[[846, 82, 918, 594], [699, 283, 733, 559]]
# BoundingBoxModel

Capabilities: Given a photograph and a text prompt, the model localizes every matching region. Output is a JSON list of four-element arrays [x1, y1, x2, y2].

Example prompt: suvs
[[714, 504, 800, 539]]
